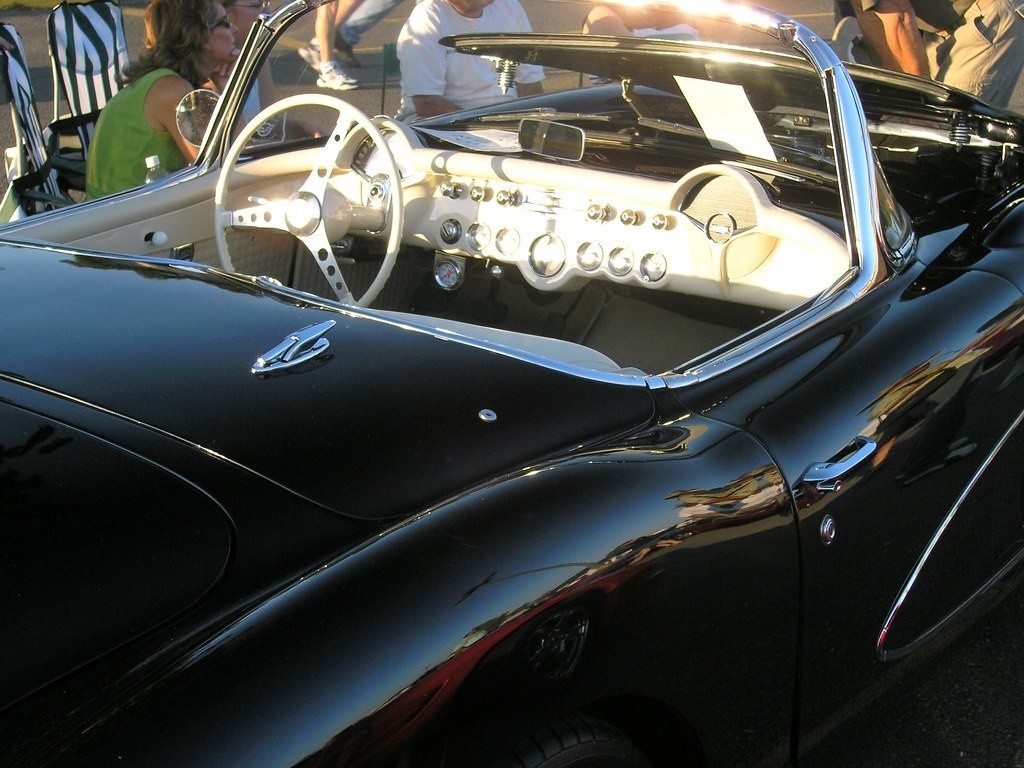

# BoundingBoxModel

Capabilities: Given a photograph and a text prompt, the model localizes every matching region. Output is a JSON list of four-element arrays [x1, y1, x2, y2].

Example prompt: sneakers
[[316, 66, 360, 91], [298, 37, 323, 72]]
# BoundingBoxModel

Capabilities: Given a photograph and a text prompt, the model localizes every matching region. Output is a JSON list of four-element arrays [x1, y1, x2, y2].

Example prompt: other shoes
[[334, 37, 356, 64]]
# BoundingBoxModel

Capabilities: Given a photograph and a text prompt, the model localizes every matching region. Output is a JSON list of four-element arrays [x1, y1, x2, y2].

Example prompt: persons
[[0, 35, 15, 51], [583, 0, 703, 87], [298, 0, 360, 92], [395, 0, 547, 127], [833, 0, 1024, 109], [85, 0, 255, 203], [332, 1, 406, 66]]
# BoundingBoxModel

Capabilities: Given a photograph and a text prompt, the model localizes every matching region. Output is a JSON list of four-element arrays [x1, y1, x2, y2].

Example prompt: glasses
[[232, 4, 263, 9], [208, 13, 231, 30]]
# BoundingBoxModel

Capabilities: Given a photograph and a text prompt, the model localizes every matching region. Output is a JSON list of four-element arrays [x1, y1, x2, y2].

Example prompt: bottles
[[144, 154, 170, 184]]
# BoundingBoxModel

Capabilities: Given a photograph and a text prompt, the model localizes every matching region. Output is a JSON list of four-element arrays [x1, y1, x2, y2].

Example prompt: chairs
[[1, 22, 81, 219], [41, 0, 135, 197]]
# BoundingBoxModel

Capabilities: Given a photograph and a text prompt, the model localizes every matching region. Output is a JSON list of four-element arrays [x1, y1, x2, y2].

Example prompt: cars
[[0, 1, 1024, 768]]
